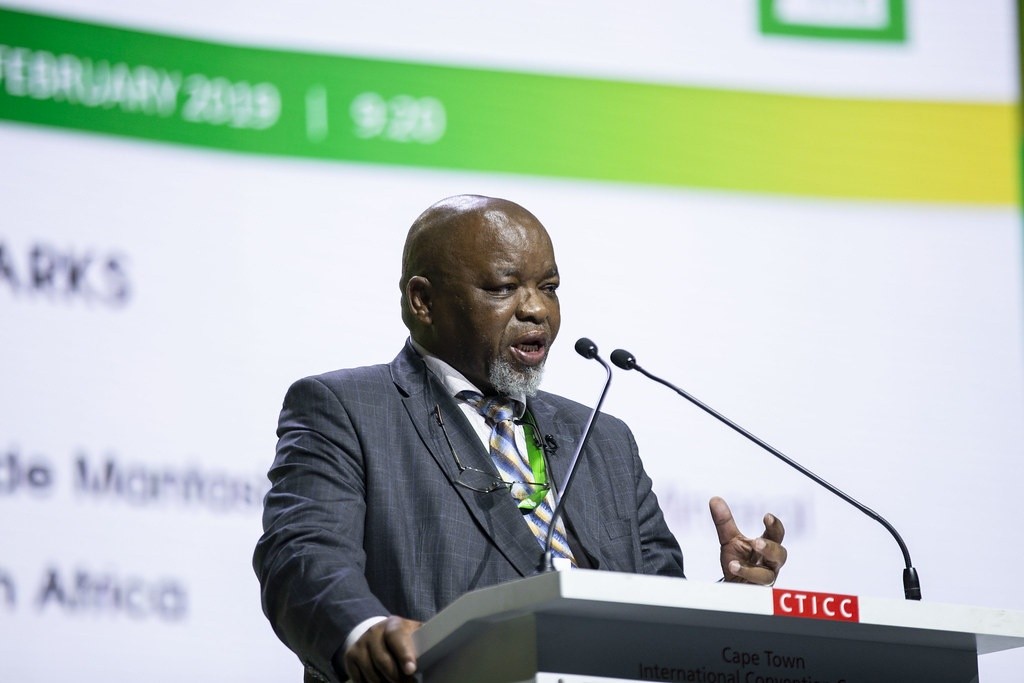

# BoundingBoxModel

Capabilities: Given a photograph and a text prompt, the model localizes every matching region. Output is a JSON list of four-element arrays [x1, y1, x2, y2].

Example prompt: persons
[[251, 194, 788, 683]]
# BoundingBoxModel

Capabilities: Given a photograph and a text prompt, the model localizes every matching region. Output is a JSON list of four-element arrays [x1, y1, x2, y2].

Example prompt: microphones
[[609, 349, 920, 601], [532, 339, 612, 574], [544, 433, 559, 455]]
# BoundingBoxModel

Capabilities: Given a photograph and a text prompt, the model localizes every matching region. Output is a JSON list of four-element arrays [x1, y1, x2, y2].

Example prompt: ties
[[457, 391, 579, 571]]
